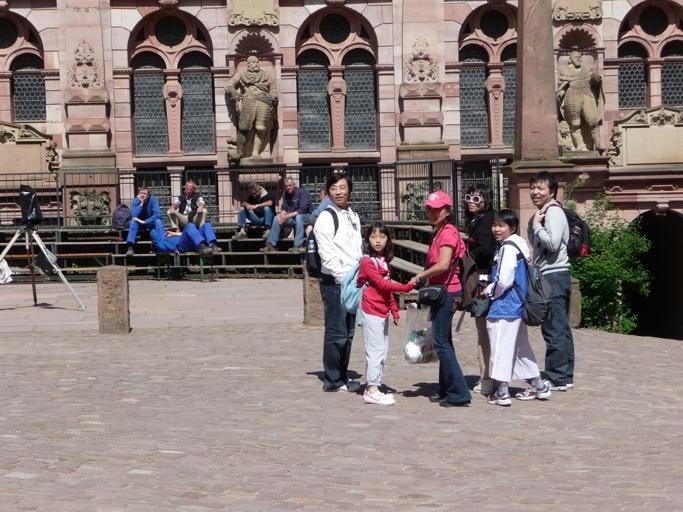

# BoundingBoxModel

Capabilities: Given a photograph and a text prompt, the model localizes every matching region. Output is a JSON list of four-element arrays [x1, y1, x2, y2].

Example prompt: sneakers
[[323, 377, 362, 392], [262, 229, 271, 238], [363, 389, 397, 406], [259, 244, 279, 252], [473, 378, 513, 406], [515, 377, 574, 400], [198, 243, 222, 254], [430, 391, 471, 407], [125, 247, 134, 257], [232, 232, 248, 240], [288, 246, 305, 253]]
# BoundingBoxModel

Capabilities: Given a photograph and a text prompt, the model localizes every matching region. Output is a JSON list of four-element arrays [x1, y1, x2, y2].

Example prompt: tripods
[[0, 226, 87, 312]]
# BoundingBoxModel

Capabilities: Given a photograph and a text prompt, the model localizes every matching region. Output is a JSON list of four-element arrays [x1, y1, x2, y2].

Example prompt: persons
[[125, 185, 166, 257], [148, 216, 224, 256], [358, 219, 422, 405], [309, 172, 365, 391], [257, 177, 316, 253], [303, 187, 333, 242], [526, 169, 577, 392], [166, 178, 208, 234], [224, 52, 279, 159], [231, 180, 275, 241], [475, 207, 552, 409], [466, 182, 499, 397], [410, 187, 473, 409], [555, 52, 608, 154]]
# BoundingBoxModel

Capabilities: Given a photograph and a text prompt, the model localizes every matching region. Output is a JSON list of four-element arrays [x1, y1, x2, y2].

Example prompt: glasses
[[464, 194, 485, 205]]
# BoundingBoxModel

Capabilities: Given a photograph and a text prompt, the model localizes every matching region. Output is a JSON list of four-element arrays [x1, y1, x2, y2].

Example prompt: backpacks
[[306, 207, 338, 278], [340, 254, 379, 314], [541, 200, 590, 258], [112, 203, 131, 229], [456, 244, 480, 311], [499, 240, 553, 326]]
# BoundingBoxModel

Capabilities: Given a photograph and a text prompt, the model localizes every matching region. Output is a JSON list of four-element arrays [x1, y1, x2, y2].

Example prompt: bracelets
[[416, 274, 420, 282]]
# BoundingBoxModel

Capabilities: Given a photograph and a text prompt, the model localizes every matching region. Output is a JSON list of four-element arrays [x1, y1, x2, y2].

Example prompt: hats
[[424, 191, 453, 209]]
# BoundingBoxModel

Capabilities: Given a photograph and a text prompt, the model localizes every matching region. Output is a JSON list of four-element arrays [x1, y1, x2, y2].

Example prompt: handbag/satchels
[[418, 285, 448, 306]]
